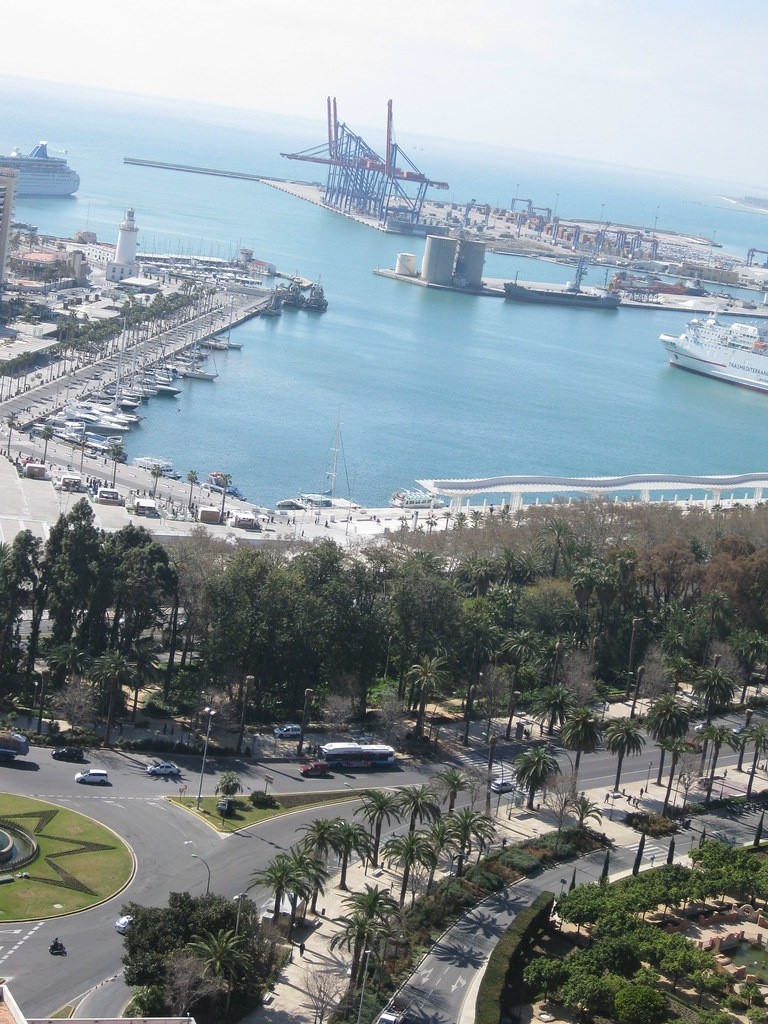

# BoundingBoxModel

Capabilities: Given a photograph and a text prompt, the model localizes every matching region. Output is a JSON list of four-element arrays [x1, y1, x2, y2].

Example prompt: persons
[[604, 787, 644, 809], [723, 769, 728, 778], [502, 837, 507, 848], [54, 938, 60, 950], [114, 723, 174, 736], [650, 855, 656, 867], [299, 942, 305, 957], [524, 724, 543, 737], [0, 449, 494, 537]]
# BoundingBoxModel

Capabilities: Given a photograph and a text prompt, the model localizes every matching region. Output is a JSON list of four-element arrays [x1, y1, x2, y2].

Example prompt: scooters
[[48, 941, 67, 956]]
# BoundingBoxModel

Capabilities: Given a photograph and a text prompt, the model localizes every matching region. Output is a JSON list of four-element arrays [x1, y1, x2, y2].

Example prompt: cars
[[490, 780, 517, 794], [694, 722, 715, 734], [50, 745, 85, 762], [113, 914, 134, 934], [273, 723, 303, 739], [675, 739, 703, 752], [145, 762, 182, 776], [732, 725, 757, 733]]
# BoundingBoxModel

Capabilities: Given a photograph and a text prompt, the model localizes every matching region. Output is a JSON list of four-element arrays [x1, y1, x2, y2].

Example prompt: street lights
[[480, 732, 504, 818], [190, 853, 211, 897], [343, 782, 373, 876], [225, 891, 249, 1013], [196, 706, 217, 811]]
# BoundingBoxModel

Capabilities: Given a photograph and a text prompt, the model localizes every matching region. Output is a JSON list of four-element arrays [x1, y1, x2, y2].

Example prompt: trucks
[[377, 996, 412, 1024], [0, 729, 30, 763]]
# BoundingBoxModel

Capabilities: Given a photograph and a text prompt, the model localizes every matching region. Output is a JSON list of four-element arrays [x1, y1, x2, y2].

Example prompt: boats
[[32, 238, 450, 513], [0, 139, 80, 198], [505, 254, 768, 393]]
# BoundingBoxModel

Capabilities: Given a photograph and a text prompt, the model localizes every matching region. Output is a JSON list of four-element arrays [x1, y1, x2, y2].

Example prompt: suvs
[[299, 760, 330, 777]]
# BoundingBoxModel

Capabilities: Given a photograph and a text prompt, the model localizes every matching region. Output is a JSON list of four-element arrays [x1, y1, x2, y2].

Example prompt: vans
[[74, 768, 109, 785]]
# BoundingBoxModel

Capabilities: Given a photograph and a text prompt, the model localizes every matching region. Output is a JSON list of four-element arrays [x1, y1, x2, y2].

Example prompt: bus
[[319, 741, 397, 770]]
